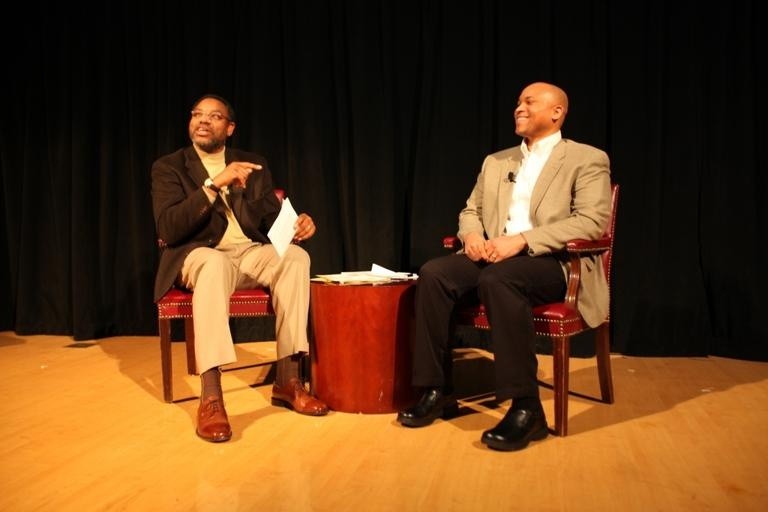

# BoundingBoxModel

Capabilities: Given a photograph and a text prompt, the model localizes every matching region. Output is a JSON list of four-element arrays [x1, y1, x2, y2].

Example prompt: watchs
[[203, 177, 222, 196]]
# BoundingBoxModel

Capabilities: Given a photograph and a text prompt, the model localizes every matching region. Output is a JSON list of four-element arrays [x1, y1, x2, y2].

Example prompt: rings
[[307, 224, 312, 231], [491, 254, 496, 259]]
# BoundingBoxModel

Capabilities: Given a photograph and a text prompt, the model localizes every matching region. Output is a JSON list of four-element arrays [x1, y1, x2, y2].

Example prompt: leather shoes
[[481, 403, 549, 451], [398, 385, 459, 427], [271, 377, 329, 415], [196, 395, 232, 441]]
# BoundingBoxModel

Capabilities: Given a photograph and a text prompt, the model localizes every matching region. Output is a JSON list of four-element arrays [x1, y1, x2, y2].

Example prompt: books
[[310, 260, 420, 289]]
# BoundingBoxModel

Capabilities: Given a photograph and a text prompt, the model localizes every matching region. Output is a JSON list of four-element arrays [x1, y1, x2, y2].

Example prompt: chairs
[[157, 188, 287, 405], [440, 179, 620, 439]]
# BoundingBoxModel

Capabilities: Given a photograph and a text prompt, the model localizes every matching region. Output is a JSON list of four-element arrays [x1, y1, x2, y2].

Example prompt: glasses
[[191, 110, 231, 122]]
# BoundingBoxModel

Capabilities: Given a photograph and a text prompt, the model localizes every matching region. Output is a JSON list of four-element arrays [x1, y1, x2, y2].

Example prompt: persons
[[149, 96, 330, 443], [398, 80, 612, 450]]
